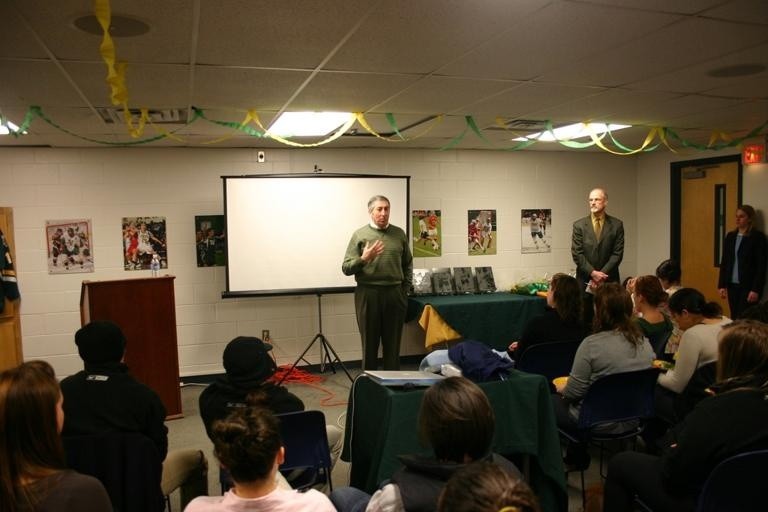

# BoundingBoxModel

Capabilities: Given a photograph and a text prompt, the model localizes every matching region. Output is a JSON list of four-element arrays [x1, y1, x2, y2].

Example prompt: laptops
[[363, 367, 445, 387]]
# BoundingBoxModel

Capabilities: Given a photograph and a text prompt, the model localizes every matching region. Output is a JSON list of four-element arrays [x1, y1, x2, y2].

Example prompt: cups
[[152, 253, 160, 278]]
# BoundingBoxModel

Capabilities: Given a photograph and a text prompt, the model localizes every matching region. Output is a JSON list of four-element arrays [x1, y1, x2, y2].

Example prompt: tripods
[[277, 299, 354, 387]]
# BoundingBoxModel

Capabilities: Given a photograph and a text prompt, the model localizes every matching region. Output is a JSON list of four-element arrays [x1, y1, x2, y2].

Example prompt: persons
[[537, 209, 547, 234], [414, 209, 440, 256], [525, 212, 550, 250], [45, 219, 90, 274], [341, 193, 414, 369], [181, 401, 339, 510], [507, 257, 768, 511], [326, 375, 527, 511], [196, 336, 346, 495], [59, 320, 211, 512], [195, 220, 227, 266], [458, 267, 471, 292], [466, 209, 495, 255], [121, 218, 168, 269], [436, 460, 543, 511], [570, 187, 626, 328], [718, 204, 768, 322], [1, 359, 115, 512], [476, 268, 490, 285]]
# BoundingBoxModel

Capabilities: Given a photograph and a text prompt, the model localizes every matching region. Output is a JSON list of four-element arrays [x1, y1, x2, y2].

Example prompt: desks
[[407, 293, 548, 353]]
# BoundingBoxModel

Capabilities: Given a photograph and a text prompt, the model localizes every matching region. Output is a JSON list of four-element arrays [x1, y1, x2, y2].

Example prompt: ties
[[595, 218, 602, 242]]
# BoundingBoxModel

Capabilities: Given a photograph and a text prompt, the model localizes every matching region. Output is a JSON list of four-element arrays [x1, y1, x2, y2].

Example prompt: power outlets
[[262, 329, 270, 342]]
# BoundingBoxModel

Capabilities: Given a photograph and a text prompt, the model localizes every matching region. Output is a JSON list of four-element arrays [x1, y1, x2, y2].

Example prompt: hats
[[74, 320, 126, 364], [222, 336, 276, 382]]
